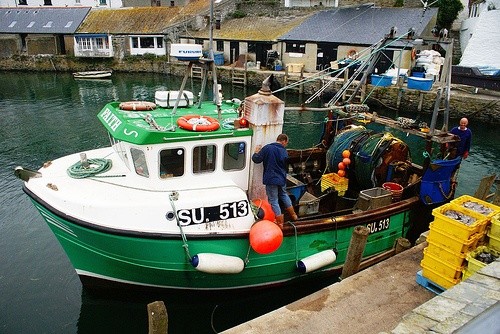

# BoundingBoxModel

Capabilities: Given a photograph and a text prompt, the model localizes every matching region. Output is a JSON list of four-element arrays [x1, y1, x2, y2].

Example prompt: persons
[[251, 133, 297, 229], [449, 118, 472, 159]]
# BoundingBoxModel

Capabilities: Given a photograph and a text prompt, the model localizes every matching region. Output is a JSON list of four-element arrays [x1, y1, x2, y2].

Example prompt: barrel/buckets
[[383, 182, 404, 203]]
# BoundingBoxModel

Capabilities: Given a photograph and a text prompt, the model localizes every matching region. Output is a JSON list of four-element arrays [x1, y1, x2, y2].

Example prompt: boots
[[285, 205, 297, 221], [274, 213, 284, 230]]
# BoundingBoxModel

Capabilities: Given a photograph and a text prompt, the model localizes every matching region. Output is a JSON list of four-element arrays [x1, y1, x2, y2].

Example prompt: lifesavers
[[177, 115, 221, 131], [119, 101, 156, 111], [347, 49, 359, 60], [411, 48, 416, 60]]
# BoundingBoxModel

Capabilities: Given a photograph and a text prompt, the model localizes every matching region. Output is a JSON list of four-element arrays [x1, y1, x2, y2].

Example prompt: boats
[[11, 83, 464, 306], [73, 69, 113, 79]]
